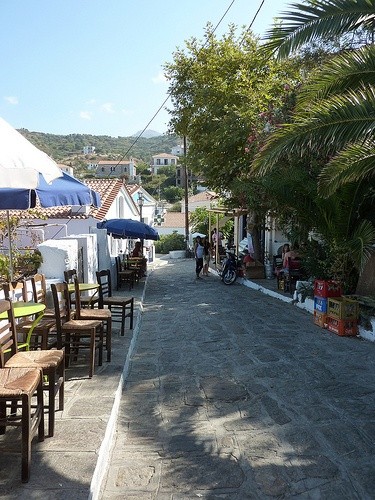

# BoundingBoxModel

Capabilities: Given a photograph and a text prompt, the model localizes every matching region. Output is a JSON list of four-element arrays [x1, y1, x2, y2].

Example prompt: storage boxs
[[245, 266, 265, 279], [294, 275, 361, 336]]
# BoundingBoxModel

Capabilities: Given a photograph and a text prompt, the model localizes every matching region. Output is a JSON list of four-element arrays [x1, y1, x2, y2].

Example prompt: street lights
[[137, 199, 144, 254]]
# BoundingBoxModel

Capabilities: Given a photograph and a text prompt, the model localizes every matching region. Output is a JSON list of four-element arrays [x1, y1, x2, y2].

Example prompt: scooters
[[219, 251, 239, 285]]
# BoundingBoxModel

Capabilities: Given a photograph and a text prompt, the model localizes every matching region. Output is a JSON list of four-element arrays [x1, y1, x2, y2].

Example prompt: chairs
[[273, 255, 308, 297], [0, 251, 147, 484]]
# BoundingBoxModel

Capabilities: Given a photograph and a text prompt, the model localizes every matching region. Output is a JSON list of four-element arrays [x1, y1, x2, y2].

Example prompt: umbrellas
[[0, 116, 102, 282], [97, 219, 159, 241]]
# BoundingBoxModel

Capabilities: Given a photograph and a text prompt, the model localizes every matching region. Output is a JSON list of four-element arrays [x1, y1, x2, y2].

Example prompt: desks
[[0, 302, 47, 353], [68, 284, 100, 309]]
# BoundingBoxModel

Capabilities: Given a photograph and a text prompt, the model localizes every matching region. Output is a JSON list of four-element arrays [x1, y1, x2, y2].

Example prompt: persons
[[281, 241, 303, 274], [212, 228, 224, 264], [193, 236, 211, 279], [133, 242, 142, 257], [226, 233, 234, 259]]
